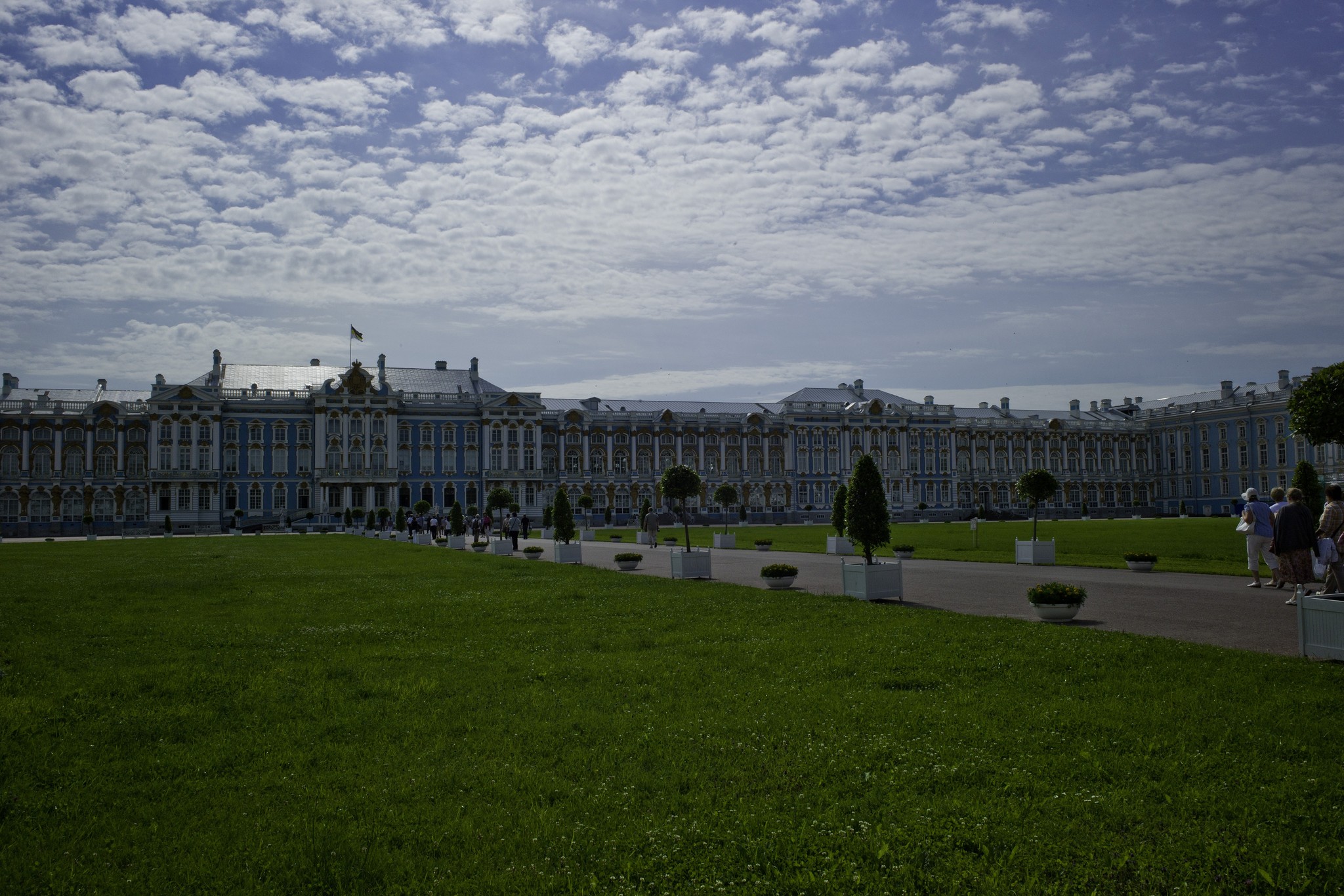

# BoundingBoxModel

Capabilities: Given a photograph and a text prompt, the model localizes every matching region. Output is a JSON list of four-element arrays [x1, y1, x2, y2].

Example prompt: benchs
[[626, 518, 640, 529], [262, 523, 289, 535], [572, 518, 591, 530], [193, 524, 222, 537]]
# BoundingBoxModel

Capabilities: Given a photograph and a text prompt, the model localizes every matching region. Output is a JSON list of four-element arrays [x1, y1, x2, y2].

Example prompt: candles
[[603, 505, 614, 529]]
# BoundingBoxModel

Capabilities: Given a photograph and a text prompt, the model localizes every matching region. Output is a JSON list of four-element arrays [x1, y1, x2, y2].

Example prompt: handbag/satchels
[[1236, 517, 1255, 535]]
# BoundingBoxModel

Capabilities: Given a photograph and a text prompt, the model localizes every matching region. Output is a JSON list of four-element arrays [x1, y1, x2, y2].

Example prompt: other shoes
[[1303, 589, 1312, 596], [1247, 582, 1261, 588], [1316, 591, 1322, 595], [1290, 585, 1294, 588], [1276, 577, 1285, 589], [1285, 599, 1297, 605], [1263, 580, 1278, 587]]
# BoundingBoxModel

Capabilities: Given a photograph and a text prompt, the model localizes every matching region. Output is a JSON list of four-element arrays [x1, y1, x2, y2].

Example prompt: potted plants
[[773, 451, 905, 603], [637, 460, 749, 580], [917, 466, 1067, 565], [1230, 499, 1240, 518], [45, 487, 581, 565], [1080, 500, 1189, 520], [578, 493, 596, 541]]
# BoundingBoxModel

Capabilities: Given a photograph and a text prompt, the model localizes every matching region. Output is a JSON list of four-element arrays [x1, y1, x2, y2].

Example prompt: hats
[[1241, 487, 1260, 501]]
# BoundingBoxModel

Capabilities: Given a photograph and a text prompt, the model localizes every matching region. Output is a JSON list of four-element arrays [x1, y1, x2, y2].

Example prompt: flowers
[[609, 534, 623, 539], [1122, 550, 1159, 562], [753, 538, 773, 545], [1024, 579, 1088, 607], [435, 537, 448, 542], [523, 545, 545, 553], [613, 553, 644, 562], [663, 536, 678, 541], [760, 561, 799, 577], [892, 543, 914, 552]]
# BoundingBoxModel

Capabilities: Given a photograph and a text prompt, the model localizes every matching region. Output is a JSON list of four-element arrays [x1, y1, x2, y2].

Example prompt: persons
[[379, 517, 394, 535], [481, 512, 492, 543], [471, 515, 475, 536], [1273, 488, 1320, 605], [506, 511, 522, 550], [1315, 485, 1344, 594], [481, 515, 485, 538], [1263, 488, 1295, 588], [644, 507, 658, 548], [502, 514, 511, 540], [519, 514, 530, 539], [433, 503, 438, 513], [278, 505, 284, 526], [632, 514, 638, 524], [472, 514, 481, 543], [406, 513, 452, 539], [1241, 487, 1285, 588], [462, 515, 471, 542], [667, 502, 670, 508]]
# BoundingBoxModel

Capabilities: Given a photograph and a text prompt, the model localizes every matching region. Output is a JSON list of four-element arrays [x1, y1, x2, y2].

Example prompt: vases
[[893, 550, 913, 560], [664, 541, 676, 546], [1029, 601, 1080, 625], [764, 575, 796, 589], [525, 552, 542, 560], [611, 538, 622, 543], [755, 544, 772, 551], [437, 542, 448, 546], [1126, 561, 1155, 573], [616, 561, 640, 571]]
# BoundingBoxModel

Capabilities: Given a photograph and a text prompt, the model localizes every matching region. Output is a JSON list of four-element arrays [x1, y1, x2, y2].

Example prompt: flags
[[351, 325, 363, 342]]
